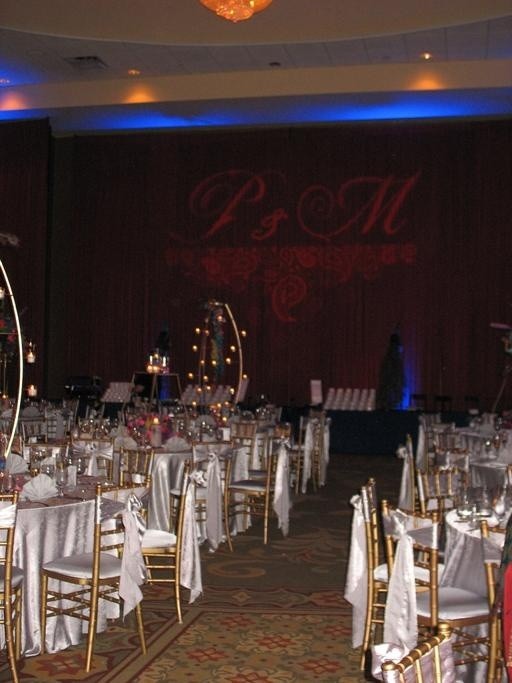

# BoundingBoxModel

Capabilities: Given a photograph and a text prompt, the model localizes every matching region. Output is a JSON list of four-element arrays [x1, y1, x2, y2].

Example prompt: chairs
[[504, 462, 512, 491], [70, 436, 115, 483], [117, 458, 191, 623], [1, 394, 286, 442], [24, 441, 71, 475], [40, 481, 149, 671], [417, 408, 512, 455], [170, 439, 235, 551], [382, 533, 501, 669], [2, 394, 283, 441], [403, 433, 428, 514], [289, 413, 325, 492], [382, 620, 456, 681], [480, 520, 510, 683], [228, 432, 289, 542], [362, 476, 430, 670], [0, 489, 25, 682], [119, 445, 154, 487], [420, 452, 470, 522]]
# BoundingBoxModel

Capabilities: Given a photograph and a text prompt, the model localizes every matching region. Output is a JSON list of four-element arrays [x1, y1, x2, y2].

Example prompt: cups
[[42, 465, 55, 480], [456, 505, 473, 522]]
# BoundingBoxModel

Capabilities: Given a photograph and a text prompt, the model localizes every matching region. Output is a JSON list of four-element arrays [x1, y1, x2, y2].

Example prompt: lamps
[[198, 0, 272, 22], [23, 384, 37, 396], [22, 348, 35, 363], [144, 346, 169, 373]]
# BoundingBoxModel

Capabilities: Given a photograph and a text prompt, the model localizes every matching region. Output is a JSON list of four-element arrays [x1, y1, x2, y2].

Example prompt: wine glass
[[217, 430, 224, 444], [76, 458, 91, 486], [2, 475, 13, 503], [56, 469, 67, 498], [63, 456, 75, 470]]
